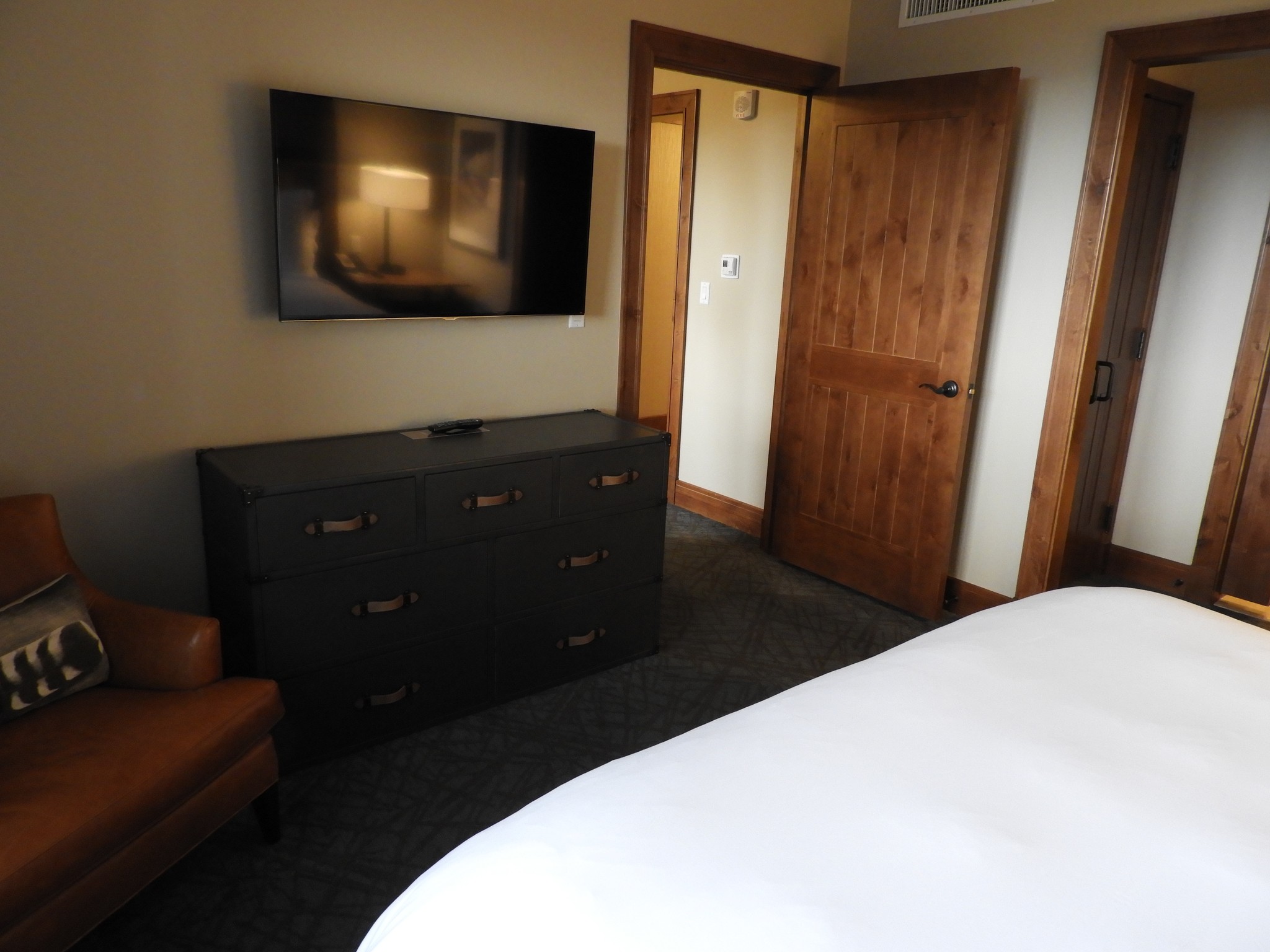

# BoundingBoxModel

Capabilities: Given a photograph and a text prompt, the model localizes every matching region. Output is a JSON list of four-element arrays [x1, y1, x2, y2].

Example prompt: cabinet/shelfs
[[199, 409, 671, 777]]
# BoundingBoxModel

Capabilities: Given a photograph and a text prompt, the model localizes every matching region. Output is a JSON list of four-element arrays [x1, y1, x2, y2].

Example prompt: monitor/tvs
[[268, 88, 596, 322]]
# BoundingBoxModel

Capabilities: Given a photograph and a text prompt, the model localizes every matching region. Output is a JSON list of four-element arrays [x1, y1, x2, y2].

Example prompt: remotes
[[428, 419, 484, 433]]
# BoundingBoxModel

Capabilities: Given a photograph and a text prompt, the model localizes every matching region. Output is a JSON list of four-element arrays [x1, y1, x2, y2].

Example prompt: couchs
[[0, 493, 284, 952]]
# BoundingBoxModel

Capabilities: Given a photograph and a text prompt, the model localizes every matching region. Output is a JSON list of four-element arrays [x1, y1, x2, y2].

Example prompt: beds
[[351, 586, 1270, 952]]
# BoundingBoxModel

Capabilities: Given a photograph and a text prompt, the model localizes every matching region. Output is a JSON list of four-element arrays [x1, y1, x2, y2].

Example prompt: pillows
[[0, 572, 108, 721]]
